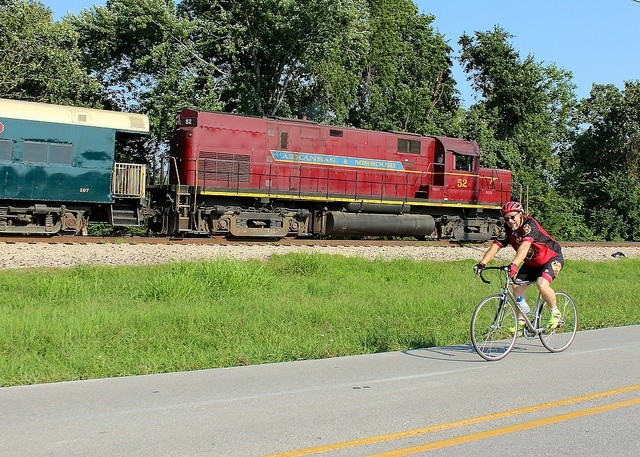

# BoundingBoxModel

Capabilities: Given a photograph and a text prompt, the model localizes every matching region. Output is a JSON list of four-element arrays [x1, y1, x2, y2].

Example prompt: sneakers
[[546, 310, 562, 330], [508, 314, 534, 335]]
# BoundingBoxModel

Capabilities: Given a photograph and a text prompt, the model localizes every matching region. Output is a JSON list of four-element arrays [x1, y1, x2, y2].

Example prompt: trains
[[0, 98, 514, 242]]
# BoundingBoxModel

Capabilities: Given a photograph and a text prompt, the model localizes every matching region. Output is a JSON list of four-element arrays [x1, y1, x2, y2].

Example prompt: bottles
[[516, 294, 530, 316]]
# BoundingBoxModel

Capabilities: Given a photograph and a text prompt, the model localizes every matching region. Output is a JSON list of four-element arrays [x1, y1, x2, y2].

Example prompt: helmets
[[501, 202, 524, 216]]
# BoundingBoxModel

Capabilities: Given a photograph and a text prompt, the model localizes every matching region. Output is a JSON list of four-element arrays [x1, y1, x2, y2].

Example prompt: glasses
[[505, 212, 519, 222]]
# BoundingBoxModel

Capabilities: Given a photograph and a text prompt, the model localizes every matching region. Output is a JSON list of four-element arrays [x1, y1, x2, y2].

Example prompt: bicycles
[[469, 267, 578, 361]]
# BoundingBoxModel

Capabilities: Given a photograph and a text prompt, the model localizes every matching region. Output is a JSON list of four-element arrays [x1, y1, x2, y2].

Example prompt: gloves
[[509, 263, 518, 278], [475, 262, 485, 276]]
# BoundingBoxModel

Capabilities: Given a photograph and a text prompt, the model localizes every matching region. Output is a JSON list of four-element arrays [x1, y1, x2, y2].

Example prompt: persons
[[472, 201, 565, 336]]
